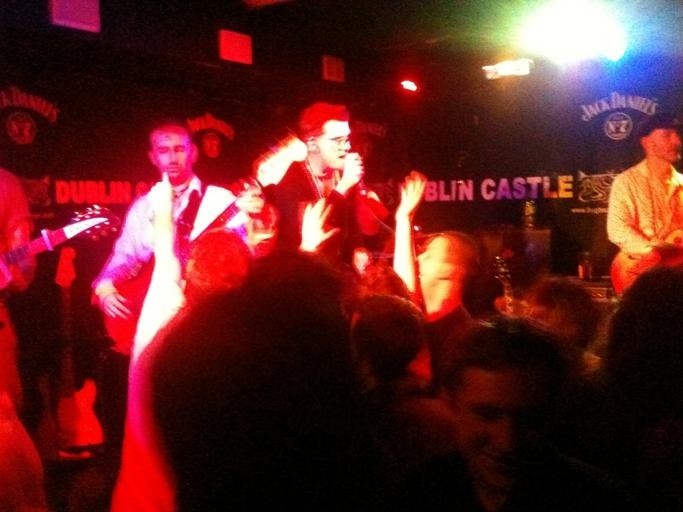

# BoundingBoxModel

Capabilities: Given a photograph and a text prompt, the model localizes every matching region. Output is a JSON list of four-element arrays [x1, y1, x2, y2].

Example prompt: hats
[[634, 112, 682, 138]]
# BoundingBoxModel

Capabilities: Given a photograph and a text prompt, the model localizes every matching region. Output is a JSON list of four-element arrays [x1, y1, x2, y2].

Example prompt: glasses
[[319, 136, 352, 148]]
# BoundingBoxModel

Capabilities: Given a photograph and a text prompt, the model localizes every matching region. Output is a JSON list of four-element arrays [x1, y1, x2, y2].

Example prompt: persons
[[0, 101, 681, 510]]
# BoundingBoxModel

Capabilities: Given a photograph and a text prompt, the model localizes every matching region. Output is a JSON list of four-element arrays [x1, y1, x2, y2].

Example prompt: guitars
[[29, 248, 108, 461], [102, 136, 308, 355], [609, 225, 683, 298]]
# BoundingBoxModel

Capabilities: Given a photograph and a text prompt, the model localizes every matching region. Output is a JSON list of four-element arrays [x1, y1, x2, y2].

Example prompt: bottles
[[522, 201, 537, 232], [577, 253, 593, 280]]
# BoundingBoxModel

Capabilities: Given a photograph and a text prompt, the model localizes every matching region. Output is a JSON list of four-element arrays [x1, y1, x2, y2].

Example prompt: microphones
[[355, 177, 367, 196]]
